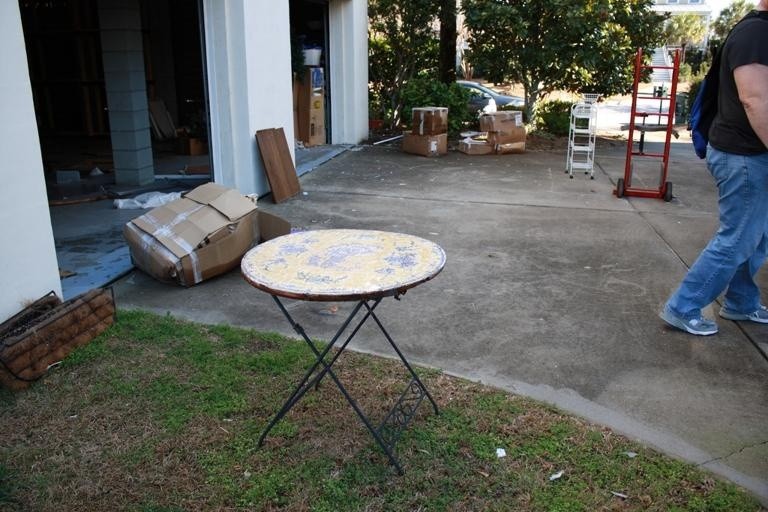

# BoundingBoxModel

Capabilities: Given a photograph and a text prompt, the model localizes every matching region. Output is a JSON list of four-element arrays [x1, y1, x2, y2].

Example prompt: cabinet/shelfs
[[565, 102, 598, 180]]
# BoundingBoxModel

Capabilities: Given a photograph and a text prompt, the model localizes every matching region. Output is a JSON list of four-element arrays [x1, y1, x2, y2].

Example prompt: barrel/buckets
[[301, 49, 321, 66]]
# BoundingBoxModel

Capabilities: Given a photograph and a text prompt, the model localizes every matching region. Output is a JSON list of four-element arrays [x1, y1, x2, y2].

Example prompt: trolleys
[[613, 46, 682, 202]]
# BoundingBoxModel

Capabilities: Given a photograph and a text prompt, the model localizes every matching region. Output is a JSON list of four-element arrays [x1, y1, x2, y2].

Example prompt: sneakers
[[659, 303, 719, 335], [719, 300, 768, 323]]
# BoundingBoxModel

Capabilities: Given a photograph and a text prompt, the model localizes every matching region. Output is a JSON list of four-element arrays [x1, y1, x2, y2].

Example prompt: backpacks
[[688, 41, 725, 158]]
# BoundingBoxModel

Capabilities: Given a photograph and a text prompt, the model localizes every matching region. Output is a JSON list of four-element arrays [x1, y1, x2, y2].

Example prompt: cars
[[456, 80, 537, 123]]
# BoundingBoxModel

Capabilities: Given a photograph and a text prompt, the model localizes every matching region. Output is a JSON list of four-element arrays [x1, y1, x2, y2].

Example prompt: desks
[[239, 228, 446, 476]]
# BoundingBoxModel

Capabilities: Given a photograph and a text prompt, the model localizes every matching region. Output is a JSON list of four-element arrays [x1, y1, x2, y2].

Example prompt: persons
[[659, 0, 768, 337]]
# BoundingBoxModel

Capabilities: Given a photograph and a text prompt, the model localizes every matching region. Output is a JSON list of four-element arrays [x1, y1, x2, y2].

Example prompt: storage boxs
[[412, 106, 448, 135], [500, 142, 526, 153], [122, 183, 291, 288], [304, 67, 324, 146], [488, 126, 526, 144], [403, 130, 446, 156], [480, 110, 521, 132], [458, 140, 492, 155]]
